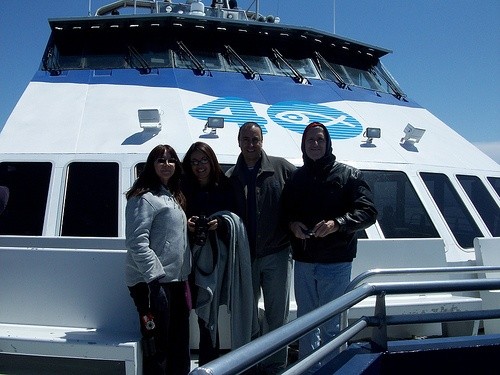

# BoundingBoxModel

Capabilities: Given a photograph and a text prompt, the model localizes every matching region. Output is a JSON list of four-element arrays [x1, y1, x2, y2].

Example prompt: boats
[[1, 0, 500, 375]]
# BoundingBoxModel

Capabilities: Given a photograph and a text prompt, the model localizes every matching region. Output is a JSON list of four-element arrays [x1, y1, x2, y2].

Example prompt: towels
[[193, 211, 260, 349]]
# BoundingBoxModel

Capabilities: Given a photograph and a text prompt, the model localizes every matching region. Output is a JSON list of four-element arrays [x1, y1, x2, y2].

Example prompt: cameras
[[191, 216, 212, 245], [141, 314, 156, 332], [304, 229, 316, 235]]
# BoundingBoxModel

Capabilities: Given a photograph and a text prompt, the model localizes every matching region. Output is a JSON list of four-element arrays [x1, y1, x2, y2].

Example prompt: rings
[[301, 233, 304, 237]]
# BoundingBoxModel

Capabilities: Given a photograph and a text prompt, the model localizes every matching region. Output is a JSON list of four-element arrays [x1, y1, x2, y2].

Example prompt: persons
[[275, 121, 377, 375], [125, 122, 300, 375]]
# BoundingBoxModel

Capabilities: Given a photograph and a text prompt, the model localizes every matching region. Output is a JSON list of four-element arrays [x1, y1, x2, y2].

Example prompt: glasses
[[190, 156, 210, 165], [156, 158, 178, 166]]
